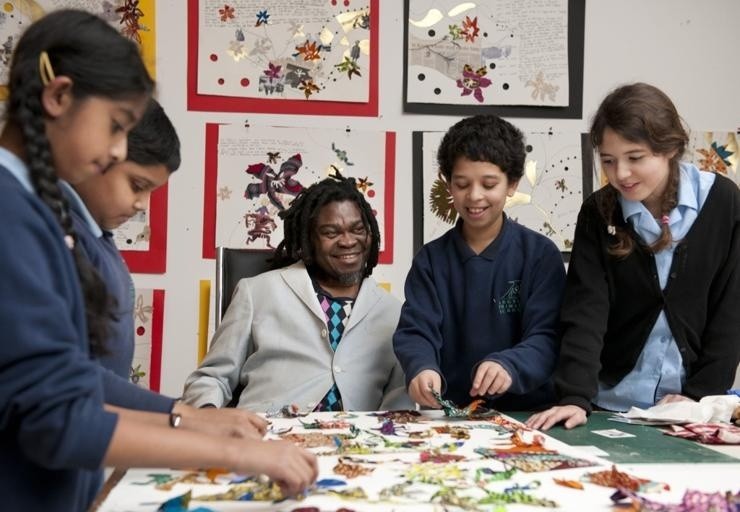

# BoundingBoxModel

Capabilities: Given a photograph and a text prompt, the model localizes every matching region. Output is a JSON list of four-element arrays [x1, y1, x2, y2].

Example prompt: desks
[[86, 411, 740, 512]]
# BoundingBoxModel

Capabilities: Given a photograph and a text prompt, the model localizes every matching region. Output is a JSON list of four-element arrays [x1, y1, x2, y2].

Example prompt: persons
[[181, 175, 418, 411], [392, 112, 567, 414], [523, 82, 740, 432]]
